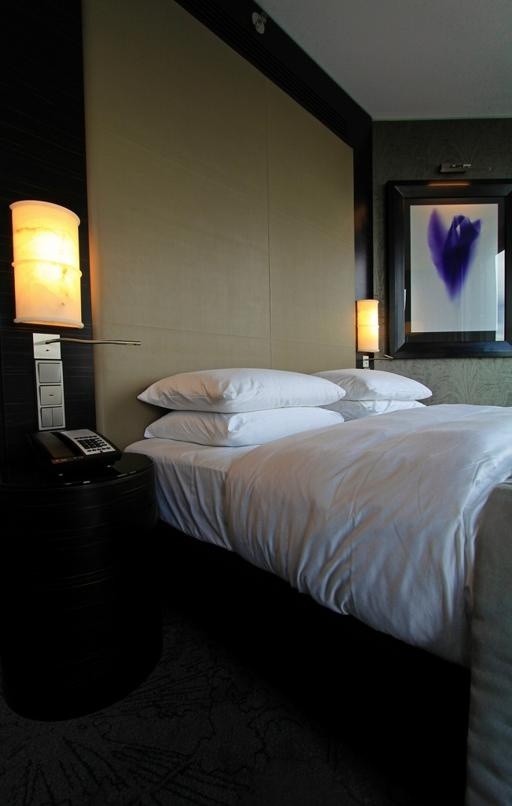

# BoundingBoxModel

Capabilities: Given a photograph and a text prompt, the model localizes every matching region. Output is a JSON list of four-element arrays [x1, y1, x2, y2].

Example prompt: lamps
[[6, 197, 88, 332], [355, 297, 382, 370]]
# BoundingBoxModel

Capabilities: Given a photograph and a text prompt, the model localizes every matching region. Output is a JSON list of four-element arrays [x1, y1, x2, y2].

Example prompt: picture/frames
[[382, 177, 510, 360]]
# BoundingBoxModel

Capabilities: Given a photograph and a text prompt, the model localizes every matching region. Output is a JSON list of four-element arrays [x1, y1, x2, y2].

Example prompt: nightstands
[[3, 452, 155, 724]]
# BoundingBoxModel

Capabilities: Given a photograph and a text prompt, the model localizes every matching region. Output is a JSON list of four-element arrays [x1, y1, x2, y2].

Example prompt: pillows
[[136, 363, 434, 448]]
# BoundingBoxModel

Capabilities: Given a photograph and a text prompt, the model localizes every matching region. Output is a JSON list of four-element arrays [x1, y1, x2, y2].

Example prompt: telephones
[[34, 428, 121, 470]]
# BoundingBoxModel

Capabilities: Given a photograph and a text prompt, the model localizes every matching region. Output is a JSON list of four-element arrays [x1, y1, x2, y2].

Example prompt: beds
[[119, 401, 511, 806]]
